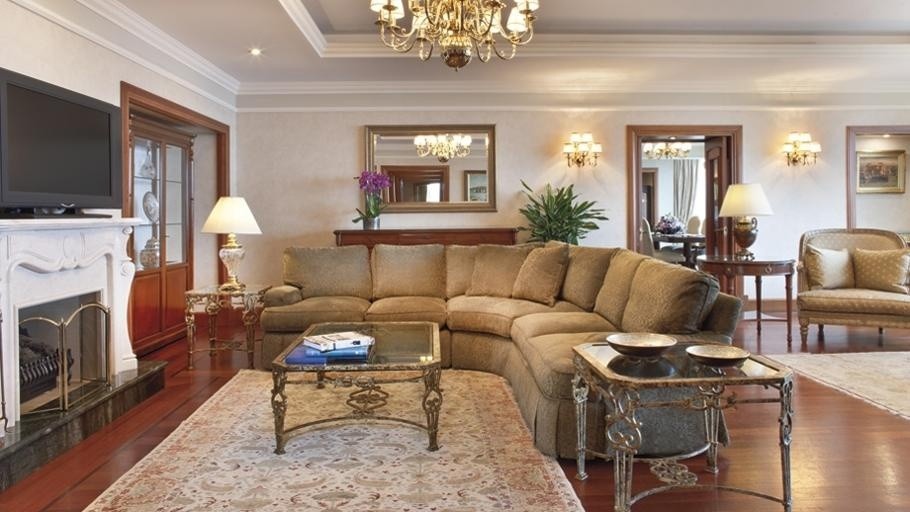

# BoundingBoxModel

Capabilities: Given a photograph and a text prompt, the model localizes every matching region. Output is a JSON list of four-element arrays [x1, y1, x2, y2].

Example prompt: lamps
[[717, 181, 775, 261], [779, 129, 821, 171], [407, 134, 474, 166], [563, 129, 605, 168], [203, 195, 262, 292], [643, 141, 694, 161]]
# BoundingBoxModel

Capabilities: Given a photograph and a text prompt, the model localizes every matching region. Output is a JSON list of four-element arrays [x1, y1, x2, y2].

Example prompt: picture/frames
[[461, 170, 488, 203], [855, 148, 906, 194]]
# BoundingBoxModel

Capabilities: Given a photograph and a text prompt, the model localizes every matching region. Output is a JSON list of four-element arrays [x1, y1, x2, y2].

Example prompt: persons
[[862, 161, 889, 184]]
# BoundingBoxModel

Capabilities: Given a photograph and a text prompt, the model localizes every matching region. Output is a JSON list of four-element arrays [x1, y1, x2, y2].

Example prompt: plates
[[686, 344, 751, 367], [142, 191, 159, 223]]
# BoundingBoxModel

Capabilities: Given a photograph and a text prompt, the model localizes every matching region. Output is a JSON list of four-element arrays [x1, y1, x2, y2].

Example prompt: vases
[[361, 217, 382, 232]]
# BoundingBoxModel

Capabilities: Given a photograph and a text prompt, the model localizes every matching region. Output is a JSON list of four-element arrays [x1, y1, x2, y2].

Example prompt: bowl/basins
[[606, 332, 679, 358], [606, 354, 678, 379]]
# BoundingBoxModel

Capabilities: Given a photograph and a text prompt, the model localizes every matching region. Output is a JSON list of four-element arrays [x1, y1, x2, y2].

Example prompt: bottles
[[140, 141, 156, 178]]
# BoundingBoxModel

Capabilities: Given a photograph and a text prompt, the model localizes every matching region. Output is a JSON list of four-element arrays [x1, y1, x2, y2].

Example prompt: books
[[303, 329, 376, 353], [285, 346, 330, 364], [304, 344, 371, 358]]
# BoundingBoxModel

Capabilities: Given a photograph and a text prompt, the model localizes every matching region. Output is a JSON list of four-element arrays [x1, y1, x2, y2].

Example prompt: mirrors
[[362, 123, 500, 217]]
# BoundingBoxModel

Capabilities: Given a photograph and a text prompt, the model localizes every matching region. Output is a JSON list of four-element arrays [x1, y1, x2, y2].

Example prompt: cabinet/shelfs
[[122, 110, 200, 360], [330, 226, 519, 250]]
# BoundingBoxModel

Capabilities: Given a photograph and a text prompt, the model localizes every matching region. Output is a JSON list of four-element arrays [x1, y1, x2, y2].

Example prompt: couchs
[[260, 239, 743, 465]]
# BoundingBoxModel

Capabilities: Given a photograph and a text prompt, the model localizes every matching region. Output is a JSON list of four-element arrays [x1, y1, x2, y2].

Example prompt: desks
[[694, 257, 796, 347], [183, 283, 273, 373], [567, 337, 796, 512], [653, 231, 713, 270]]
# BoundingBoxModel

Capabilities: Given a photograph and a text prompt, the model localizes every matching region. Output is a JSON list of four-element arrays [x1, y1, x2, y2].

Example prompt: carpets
[[74, 361, 586, 512], [750, 345, 910, 426]]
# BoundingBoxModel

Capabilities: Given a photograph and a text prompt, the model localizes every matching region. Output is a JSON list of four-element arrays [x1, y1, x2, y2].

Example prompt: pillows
[[465, 243, 532, 300], [512, 242, 571, 307], [849, 245, 910, 297], [803, 243, 860, 291]]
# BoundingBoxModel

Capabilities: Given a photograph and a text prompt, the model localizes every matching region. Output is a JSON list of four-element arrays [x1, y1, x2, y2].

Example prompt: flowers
[[656, 210, 687, 233], [354, 168, 392, 222]]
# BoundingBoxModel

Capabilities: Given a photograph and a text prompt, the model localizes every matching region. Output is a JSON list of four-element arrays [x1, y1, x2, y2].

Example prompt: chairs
[[793, 218, 910, 355], [686, 214, 699, 235], [698, 217, 707, 249], [690, 244, 709, 266], [638, 216, 685, 264]]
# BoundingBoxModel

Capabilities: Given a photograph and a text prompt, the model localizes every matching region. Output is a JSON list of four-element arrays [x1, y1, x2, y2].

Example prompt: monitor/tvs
[[0, 67, 122, 218]]
